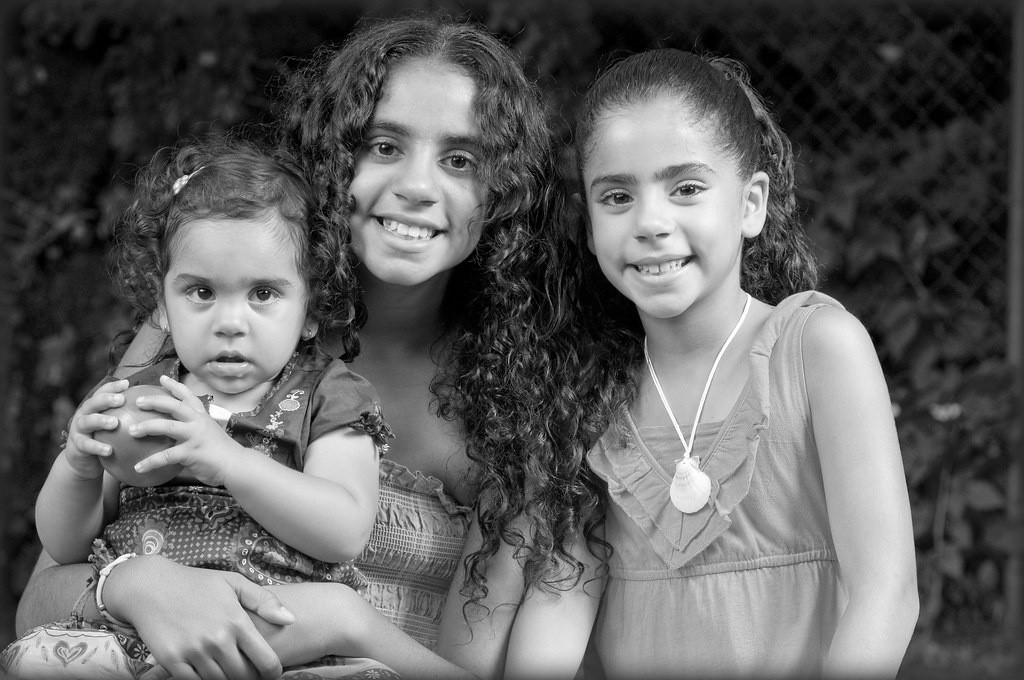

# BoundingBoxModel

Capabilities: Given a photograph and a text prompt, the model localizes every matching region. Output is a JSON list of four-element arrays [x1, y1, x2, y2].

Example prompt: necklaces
[[640, 290, 752, 515]]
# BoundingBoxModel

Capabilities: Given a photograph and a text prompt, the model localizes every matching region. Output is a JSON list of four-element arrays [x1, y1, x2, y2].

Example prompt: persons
[[33, 141, 478, 680], [7, 23, 562, 678], [502, 50, 921, 680]]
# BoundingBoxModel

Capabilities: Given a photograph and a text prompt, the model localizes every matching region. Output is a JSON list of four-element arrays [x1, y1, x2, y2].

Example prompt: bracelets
[[94, 549, 137, 632]]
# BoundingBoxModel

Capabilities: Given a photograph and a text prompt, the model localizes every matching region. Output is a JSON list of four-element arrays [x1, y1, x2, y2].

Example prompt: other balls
[[92, 383, 186, 488]]
